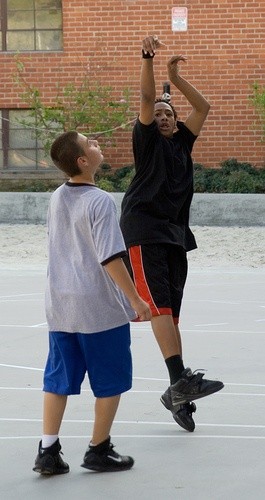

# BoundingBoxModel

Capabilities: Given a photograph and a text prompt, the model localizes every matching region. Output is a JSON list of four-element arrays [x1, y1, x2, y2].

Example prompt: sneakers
[[31, 437, 69, 474], [160, 388, 196, 432], [80, 435, 134, 472], [169, 368, 224, 405]]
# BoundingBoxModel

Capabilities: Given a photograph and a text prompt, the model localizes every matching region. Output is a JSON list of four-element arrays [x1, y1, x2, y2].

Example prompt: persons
[[24, 130, 152, 476], [120, 35, 226, 433]]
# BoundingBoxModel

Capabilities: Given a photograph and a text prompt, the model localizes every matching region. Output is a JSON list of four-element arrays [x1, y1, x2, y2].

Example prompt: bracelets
[[141, 49, 156, 59]]
